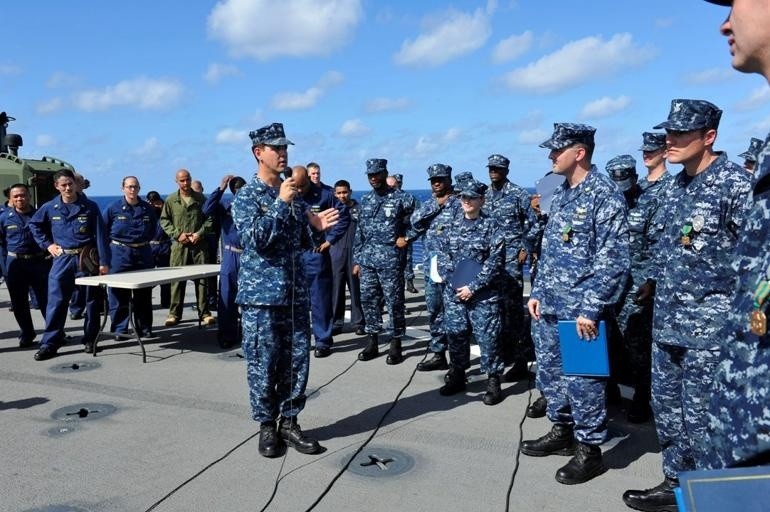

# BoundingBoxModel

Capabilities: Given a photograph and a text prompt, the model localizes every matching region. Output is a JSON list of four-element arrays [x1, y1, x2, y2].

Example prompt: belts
[[149, 239, 171, 245], [7, 250, 42, 259], [111, 239, 149, 247], [58, 248, 82, 255], [223, 244, 244, 254]]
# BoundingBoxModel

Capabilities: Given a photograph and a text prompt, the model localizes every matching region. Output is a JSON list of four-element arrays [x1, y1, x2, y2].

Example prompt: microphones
[[284, 167, 296, 216]]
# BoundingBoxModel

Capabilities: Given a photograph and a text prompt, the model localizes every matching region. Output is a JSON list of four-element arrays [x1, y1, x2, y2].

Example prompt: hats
[[538, 123, 597, 151], [736, 137, 765, 162], [652, 98, 723, 134], [604, 155, 637, 192], [364, 158, 388, 174], [638, 132, 666, 154], [454, 171, 488, 198], [393, 172, 404, 187], [249, 122, 295, 148], [486, 154, 512, 170], [425, 163, 452, 182]]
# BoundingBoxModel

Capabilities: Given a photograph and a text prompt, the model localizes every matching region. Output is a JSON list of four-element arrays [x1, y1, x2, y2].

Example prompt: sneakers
[[554, 442, 607, 485], [165, 300, 242, 348], [313, 344, 334, 357], [525, 396, 547, 419], [440, 369, 467, 395], [418, 351, 449, 371], [606, 381, 651, 423], [506, 360, 528, 382], [259, 418, 282, 459], [332, 277, 419, 336], [622, 475, 682, 511], [17, 309, 153, 360]]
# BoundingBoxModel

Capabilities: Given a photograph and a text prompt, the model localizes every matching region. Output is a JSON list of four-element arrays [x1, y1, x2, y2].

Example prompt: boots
[[279, 416, 321, 454], [484, 374, 503, 405], [386, 338, 401, 364], [520, 424, 577, 457], [358, 335, 381, 361]]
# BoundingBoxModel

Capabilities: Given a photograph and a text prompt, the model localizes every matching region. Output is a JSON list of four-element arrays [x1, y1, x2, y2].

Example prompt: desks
[[74, 262, 223, 366]]
